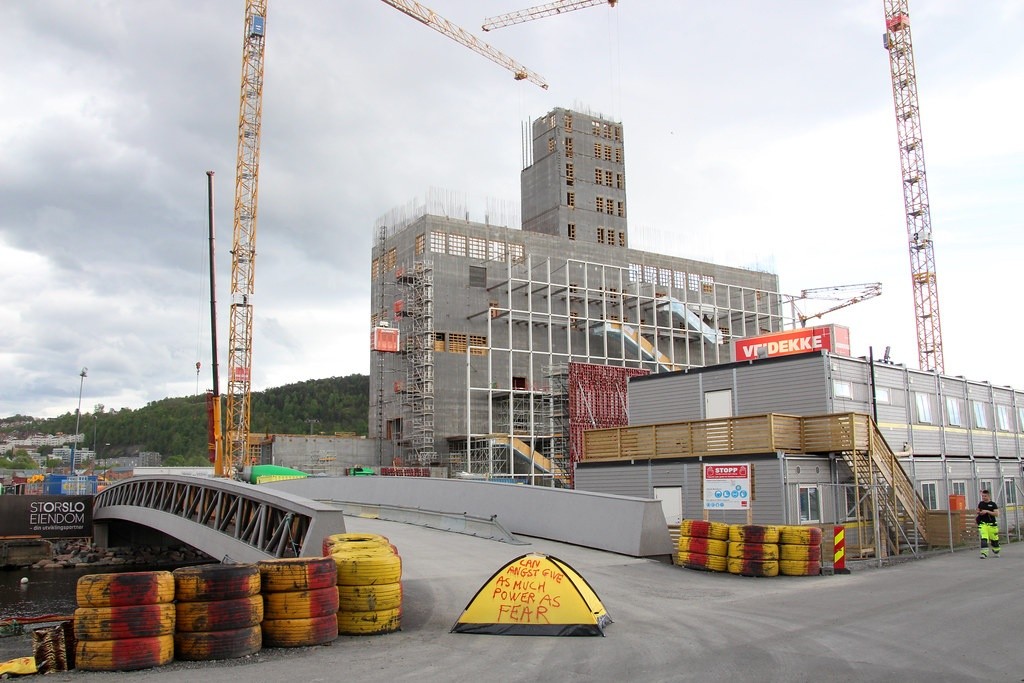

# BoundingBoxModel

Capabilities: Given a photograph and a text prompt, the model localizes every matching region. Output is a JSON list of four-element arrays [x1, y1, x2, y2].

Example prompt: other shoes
[[993, 553, 1000, 558], [980, 553, 987, 559]]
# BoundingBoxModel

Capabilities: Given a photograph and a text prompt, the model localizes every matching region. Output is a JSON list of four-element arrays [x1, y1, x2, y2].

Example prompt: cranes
[[481, 0, 946, 375], [225, 0, 547, 478]]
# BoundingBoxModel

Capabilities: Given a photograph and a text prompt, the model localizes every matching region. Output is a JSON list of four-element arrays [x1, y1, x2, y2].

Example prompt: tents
[[448, 550, 615, 638]]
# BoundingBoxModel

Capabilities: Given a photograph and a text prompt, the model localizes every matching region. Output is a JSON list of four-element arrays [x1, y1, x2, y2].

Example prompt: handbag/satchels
[[976, 515, 981, 525]]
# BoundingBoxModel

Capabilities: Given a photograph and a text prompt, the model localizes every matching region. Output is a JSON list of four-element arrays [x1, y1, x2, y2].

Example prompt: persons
[[976, 490, 1001, 560]]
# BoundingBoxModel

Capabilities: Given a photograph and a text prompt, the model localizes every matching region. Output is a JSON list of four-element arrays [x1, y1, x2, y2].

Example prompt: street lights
[[72, 366, 88, 476]]
